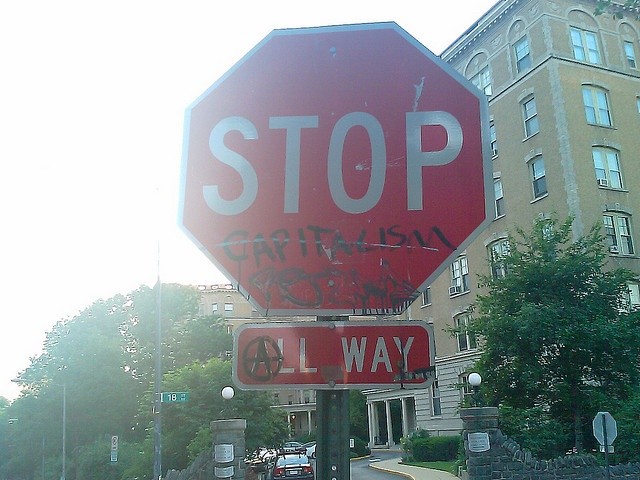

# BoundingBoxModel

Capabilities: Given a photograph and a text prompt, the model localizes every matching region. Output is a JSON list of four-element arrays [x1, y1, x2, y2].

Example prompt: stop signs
[[182, 16, 496, 323]]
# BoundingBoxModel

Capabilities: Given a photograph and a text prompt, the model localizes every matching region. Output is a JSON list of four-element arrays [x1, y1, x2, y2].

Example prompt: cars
[[244, 447, 271, 464], [278, 440, 317, 458], [272, 455, 314, 480], [272, 447, 278, 455]]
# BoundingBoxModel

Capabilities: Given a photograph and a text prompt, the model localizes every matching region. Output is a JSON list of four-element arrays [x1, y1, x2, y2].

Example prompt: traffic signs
[[161, 392, 190, 403], [232, 322, 436, 391]]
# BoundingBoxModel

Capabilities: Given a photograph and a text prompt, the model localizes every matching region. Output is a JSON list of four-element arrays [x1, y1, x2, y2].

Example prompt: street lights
[[9, 419, 46, 480], [11, 377, 67, 480]]
